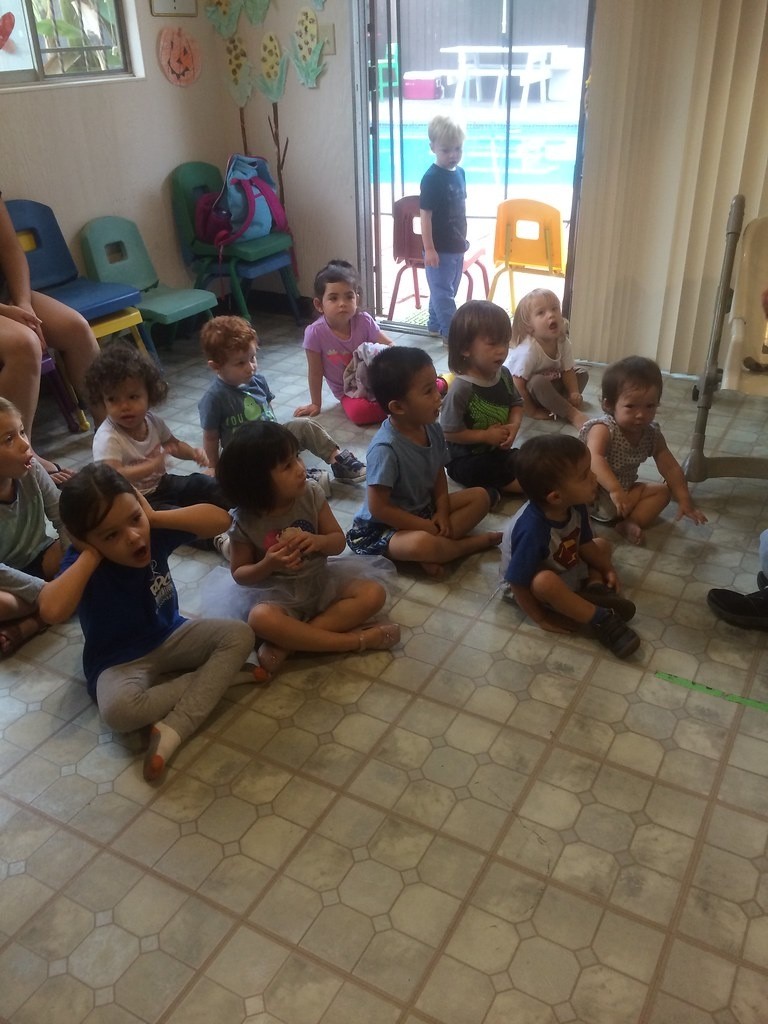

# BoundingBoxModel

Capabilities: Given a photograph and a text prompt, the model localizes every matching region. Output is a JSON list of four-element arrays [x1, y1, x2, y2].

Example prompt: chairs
[[388, 196, 490, 321], [489, 199, 566, 317], [368, 42, 398, 100], [663, 194, 768, 501], [0, 162, 307, 433]]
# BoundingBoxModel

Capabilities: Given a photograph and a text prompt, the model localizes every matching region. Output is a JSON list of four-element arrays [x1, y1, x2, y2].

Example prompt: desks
[[440, 45, 564, 106]]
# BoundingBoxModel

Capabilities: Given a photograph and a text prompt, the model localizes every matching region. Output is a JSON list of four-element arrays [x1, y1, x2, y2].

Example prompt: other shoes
[[444, 338, 450, 349], [437, 371, 456, 394], [348, 621, 401, 652], [487, 483, 504, 512], [257, 639, 295, 675], [428, 329, 441, 336]]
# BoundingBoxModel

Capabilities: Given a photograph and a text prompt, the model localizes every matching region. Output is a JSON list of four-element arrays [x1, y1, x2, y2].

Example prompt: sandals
[[43, 464, 79, 488], [0, 611, 53, 656]]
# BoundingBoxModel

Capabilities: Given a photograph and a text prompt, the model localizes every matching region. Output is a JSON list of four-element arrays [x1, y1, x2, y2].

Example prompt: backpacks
[[197, 153, 291, 248]]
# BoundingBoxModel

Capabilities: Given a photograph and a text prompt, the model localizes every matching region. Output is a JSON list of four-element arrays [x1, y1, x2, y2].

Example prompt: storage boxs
[[402, 70, 444, 100]]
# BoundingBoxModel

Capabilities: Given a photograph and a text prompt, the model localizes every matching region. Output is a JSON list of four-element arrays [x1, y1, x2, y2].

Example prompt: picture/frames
[[150, 0, 199, 17]]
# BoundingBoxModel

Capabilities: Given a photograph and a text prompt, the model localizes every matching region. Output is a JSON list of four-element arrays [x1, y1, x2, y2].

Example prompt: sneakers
[[573, 588, 636, 621], [591, 612, 640, 659], [708, 571, 768, 632], [305, 468, 331, 499], [213, 531, 232, 562], [333, 448, 368, 484]]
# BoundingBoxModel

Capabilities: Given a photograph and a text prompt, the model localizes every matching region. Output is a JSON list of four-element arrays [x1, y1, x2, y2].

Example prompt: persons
[[217, 421, 401, 680], [83, 347, 234, 563], [504, 290, 589, 429], [578, 355, 709, 545], [441, 301, 525, 512], [0, 395, 70, 654], [500, 433, 640, 657], [0, 197, 107, 488], [420, 114, 470, 345], [198, 319, 368, 496], [38, 464, 269, 780], [706, 528, 768, 628], [346, 345, 503, 574], [292, 261, 456, 425]]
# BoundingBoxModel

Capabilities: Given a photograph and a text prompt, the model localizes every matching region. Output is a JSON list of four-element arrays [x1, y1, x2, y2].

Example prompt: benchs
[[441, 64, 548, 104]]
